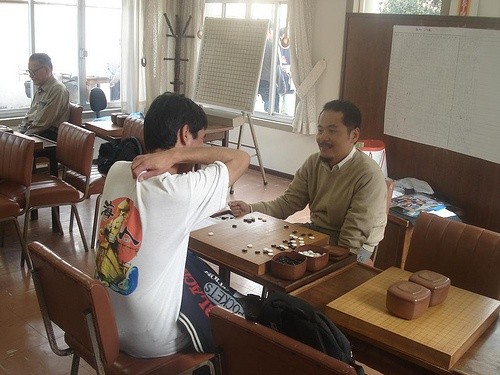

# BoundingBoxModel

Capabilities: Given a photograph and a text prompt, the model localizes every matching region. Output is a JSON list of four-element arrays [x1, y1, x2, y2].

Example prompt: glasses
[[26, 66, 45, 76]]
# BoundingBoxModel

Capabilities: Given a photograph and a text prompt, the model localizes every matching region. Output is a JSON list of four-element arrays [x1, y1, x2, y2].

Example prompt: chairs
[[0, 129, 35, 269], [69, 116, 146, 248], [27, 241, 216, 375], [209, 306, 357, 375], [70, 103, 83, 127], [0, 122, 95, 268], [404, 212, 500, 301]]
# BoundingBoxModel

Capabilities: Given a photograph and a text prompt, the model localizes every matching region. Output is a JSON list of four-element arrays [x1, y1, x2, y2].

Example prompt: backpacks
[[249, 292, 368, 375]]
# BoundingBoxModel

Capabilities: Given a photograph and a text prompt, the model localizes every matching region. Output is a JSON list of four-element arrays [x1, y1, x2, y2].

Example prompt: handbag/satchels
[[98, 136, 143, 176]]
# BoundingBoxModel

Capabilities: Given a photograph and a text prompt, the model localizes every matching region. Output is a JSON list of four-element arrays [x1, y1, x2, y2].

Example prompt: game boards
[[189, 209, 330, 277], [84, 121, 123, 138], [325, 265, 500, 371]]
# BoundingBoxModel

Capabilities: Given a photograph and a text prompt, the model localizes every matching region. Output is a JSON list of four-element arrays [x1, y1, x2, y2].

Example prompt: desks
[[289, 262, 500, 375], [374, 208, 465, 271], [10, 126, 59, 232], [187, 246, 357, 299], [81, 124, 234, 194]]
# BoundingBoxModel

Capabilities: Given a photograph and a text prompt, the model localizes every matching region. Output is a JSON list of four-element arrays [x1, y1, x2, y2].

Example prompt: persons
[[258, 39, 280, 114], [94, 91, 253, 359], [229, 100, 387, 296], [14, 53, 70, 143]]
